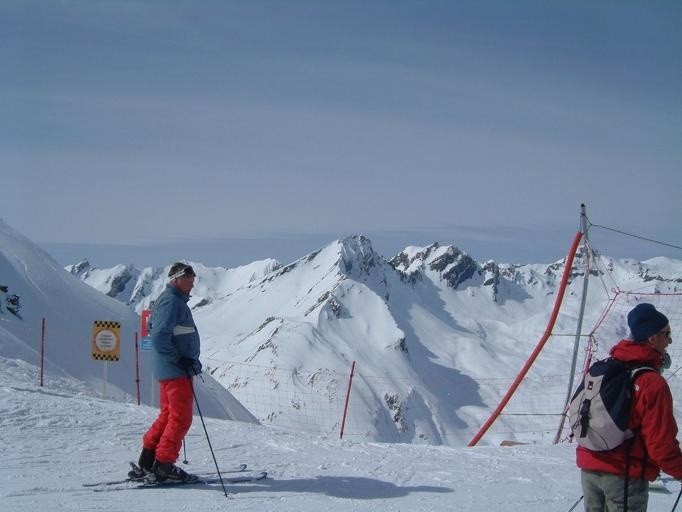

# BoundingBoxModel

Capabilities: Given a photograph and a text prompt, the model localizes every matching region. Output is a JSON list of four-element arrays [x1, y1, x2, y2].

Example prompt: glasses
[[170, 265, 193, 282]]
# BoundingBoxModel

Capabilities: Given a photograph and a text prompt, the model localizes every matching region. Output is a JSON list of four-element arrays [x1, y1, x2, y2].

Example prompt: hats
[[628, 303, 670, 341]]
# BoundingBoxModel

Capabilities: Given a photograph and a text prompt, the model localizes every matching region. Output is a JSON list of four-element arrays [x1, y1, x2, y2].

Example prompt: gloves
[[173, 356, 196, 371]]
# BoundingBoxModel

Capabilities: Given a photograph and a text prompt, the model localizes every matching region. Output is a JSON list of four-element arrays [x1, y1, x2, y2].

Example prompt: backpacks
[[566, 357, 654, 451]]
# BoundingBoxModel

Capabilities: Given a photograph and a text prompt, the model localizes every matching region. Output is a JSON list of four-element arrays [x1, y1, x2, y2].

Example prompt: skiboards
[[81, 463, 267, 492]]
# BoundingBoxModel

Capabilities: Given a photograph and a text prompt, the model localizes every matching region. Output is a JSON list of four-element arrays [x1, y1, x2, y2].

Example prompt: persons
[[138, 262, 203, 483], [575, 303, 682, 512]]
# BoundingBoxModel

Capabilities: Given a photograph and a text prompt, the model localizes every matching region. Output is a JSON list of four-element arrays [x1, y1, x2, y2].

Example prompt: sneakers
[[139, 449, 198, 483]]
